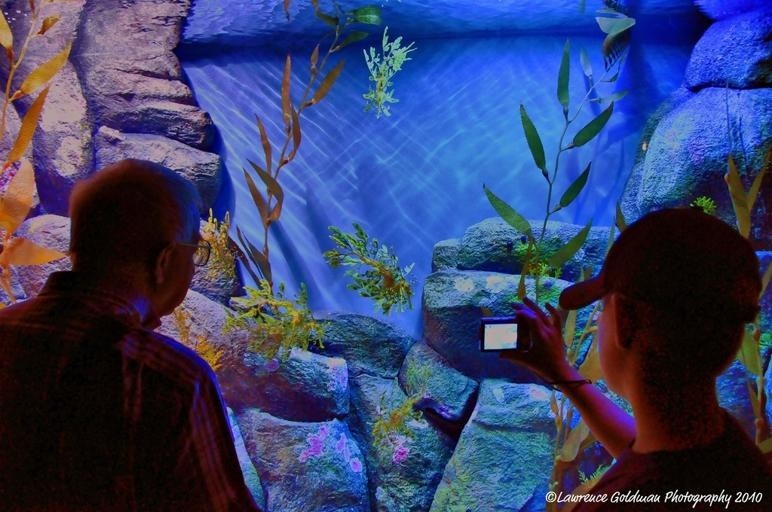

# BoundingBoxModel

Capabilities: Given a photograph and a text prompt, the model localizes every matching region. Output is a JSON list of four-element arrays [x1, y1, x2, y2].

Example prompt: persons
[[499, 204, 770, 511], [0, 159, 261, 512]]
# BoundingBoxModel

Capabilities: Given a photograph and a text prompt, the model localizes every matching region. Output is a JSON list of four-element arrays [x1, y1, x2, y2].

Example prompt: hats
[[558, 204, 763, 322]]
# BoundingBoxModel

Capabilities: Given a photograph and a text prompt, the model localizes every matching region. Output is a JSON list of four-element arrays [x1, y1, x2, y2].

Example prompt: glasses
[[160, 236, 211, 267]]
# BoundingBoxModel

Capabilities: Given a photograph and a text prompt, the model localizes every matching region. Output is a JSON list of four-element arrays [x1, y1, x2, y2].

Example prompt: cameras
[[480, 316, 534, 352]]
